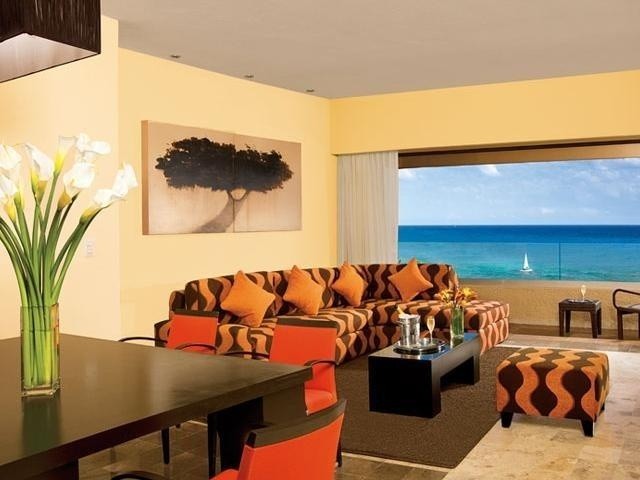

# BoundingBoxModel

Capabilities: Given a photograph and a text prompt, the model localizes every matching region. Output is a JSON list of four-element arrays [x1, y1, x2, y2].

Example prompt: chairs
[[613, 289, 640, 340], [112, 310, 347, 480]]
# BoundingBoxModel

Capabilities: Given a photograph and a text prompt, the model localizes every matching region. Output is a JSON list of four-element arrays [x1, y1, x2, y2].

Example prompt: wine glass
[[426, 315, 436, 345], [580, 285, 587, 303]]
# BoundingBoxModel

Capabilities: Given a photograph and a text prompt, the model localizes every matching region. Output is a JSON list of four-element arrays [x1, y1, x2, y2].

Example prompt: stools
[[497, 347, 610, 436]]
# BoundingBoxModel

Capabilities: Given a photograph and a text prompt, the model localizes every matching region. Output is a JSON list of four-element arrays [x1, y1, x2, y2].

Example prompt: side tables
[[559, 298, 601, 338]]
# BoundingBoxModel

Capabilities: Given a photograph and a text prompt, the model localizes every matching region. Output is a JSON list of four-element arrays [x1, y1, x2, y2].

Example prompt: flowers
[[0, 130, 138, 390], [433, 285, 480, 335]]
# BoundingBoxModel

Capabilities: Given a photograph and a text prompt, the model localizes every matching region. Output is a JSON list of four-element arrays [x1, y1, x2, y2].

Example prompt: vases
[[450, 307, 464, 339], [19, 304, 61, 397]]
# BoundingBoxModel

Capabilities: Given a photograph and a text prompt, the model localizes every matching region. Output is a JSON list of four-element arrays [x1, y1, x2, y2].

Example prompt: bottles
[[399, 313, 421, 348]]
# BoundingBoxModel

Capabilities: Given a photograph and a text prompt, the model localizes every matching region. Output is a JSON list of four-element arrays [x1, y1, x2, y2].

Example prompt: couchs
[[153, 264, 510, 366]]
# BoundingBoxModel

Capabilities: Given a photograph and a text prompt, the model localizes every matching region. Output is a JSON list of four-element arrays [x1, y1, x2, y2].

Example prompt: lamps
[[1, 0, 100, 86]]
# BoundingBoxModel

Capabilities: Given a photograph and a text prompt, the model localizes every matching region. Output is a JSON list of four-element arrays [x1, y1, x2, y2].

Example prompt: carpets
[[191, 346, 519, 468]]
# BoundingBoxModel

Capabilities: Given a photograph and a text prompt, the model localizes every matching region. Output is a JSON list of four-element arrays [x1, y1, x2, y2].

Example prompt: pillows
[[221, 257, 436, 328]]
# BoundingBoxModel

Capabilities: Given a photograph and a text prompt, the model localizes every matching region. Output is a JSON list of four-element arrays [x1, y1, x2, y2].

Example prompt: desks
[[0, 335, 312, 479]]
